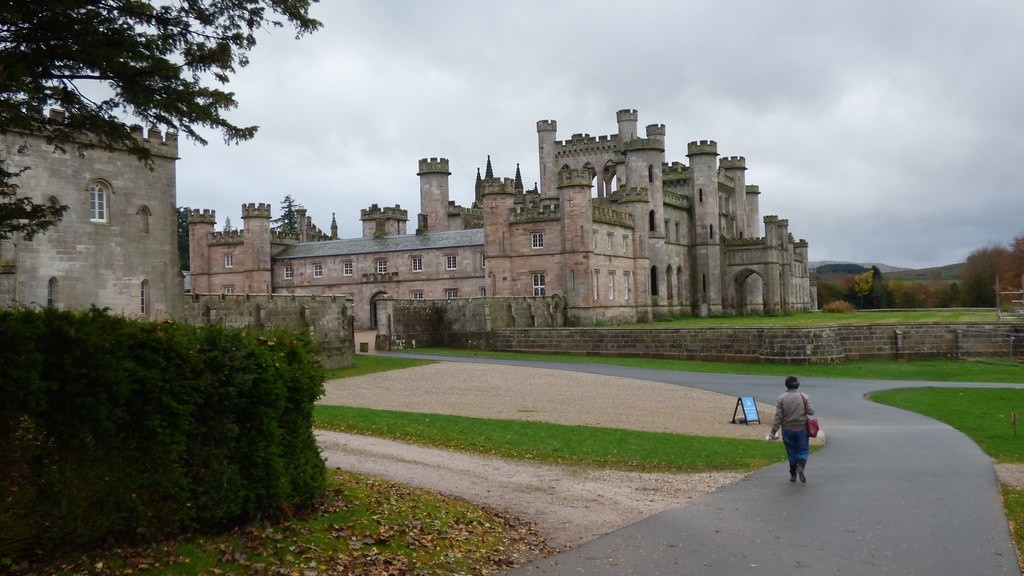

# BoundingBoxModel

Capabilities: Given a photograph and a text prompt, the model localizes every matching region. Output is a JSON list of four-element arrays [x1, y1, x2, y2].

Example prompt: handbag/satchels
[[807, 418, 819, 438]]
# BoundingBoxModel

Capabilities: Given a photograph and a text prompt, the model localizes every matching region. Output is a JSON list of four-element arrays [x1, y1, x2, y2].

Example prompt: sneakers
[[795, 466, 806, 482], [790, 476, 796, 482]]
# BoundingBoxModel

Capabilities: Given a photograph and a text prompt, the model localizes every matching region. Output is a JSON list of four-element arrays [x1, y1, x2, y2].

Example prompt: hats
[[784, 375, 800, 388]]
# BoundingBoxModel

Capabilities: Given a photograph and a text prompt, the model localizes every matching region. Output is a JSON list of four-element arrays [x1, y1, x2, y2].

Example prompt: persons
[[770, 374, 815, 483]]
